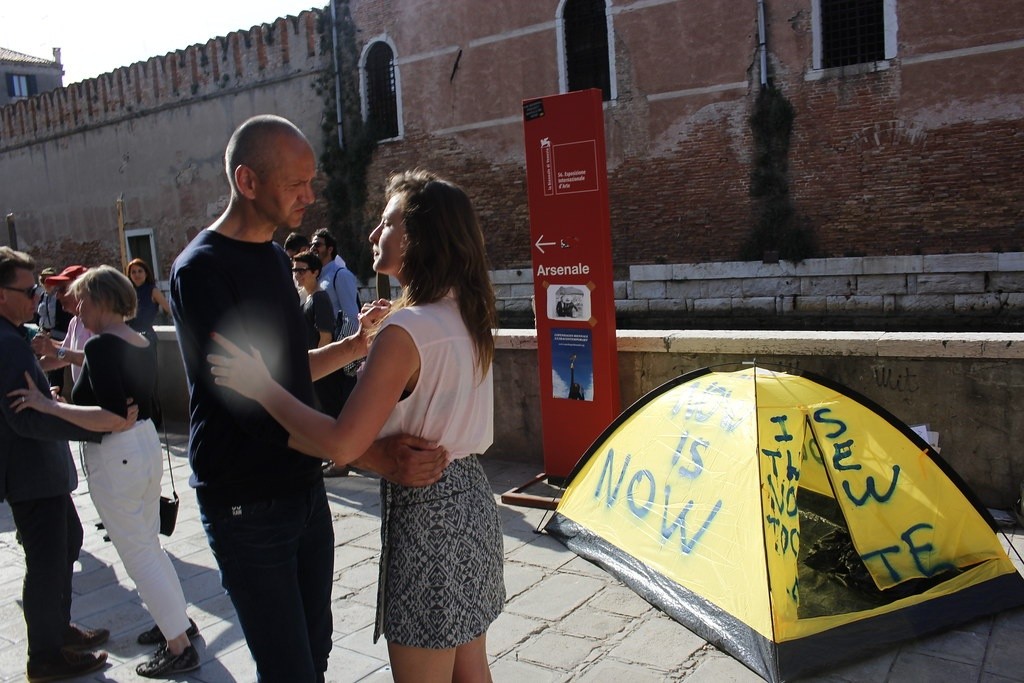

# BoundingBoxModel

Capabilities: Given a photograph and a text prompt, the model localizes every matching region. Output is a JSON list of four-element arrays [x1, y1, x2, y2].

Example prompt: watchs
[[58, 347, 65, 360]]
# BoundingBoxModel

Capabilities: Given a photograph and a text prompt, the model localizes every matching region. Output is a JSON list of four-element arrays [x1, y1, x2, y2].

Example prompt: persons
[[569, 362, 585, 400], [556, 297, 578, 317], [169, 114, 451, 683], [283, 228, 364, 479], [207, 170, 506, 683], [0, 245, 200, 681]]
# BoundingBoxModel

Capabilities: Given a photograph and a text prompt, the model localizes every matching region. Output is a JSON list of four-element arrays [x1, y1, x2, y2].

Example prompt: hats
[[43, 265, 90, 290], [41, 267, 59, 276]]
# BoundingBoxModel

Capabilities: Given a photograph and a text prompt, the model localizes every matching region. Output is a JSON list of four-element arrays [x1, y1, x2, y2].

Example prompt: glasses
[[291, 267, 311, 273], [1, 284, 38, 299], [54, 285, 66, 293], [40, 278, 47, 284], [309, 241, 326, 248]]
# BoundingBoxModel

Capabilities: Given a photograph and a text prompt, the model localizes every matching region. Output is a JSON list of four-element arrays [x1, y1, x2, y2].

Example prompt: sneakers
[[322, 461, 349, 477], [25, 647, 108, 683], [27, 622, 110, 656], [136, 640, 201, 677], [136, 616, 200, 644]]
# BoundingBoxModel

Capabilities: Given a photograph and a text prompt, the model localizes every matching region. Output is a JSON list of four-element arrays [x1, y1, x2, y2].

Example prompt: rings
[[22, 396, 24, 403]]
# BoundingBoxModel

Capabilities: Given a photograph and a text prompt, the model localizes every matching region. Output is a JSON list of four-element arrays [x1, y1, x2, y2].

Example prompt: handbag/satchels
[[330, 267, 368, 377], [159, 492, 180, 537]]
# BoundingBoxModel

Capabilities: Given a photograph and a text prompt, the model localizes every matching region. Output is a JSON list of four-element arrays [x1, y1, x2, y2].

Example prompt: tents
[[532, 358, 1024, 682]]
